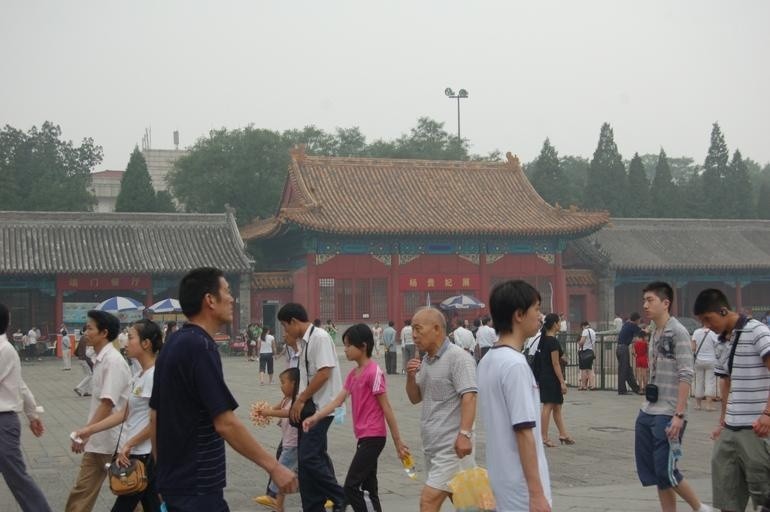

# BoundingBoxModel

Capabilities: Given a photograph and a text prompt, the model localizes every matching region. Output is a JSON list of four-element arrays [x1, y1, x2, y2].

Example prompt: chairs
[[47, 340, 57, 355]]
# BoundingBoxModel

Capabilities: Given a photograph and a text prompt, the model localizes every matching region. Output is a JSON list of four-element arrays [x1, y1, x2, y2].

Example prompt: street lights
[[444, 86, 470, 162]]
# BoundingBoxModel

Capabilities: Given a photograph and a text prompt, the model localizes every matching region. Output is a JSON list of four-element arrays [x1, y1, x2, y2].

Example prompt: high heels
[[541, 437, 556, 449], [559, 436, 575, 446]]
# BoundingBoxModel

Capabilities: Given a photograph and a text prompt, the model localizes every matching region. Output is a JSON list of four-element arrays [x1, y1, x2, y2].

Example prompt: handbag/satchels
[[443, 452, 498, 512], [287, 398, 316, 427], [105, 459, 148, 495], [577, 349, 594, 364], [523, 334, 543, 379]]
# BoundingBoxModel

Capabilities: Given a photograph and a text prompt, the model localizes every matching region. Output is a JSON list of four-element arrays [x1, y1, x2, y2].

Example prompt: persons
[[2, 267, 770, 512]]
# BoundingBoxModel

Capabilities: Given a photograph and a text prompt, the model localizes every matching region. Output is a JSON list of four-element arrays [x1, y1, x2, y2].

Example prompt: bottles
[[666, 422, 684, 460], [401, 452, 417, 480], [751, 420, 770, 445]]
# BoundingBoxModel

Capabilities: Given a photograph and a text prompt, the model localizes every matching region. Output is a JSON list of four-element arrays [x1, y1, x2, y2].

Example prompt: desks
[[36, 340, 48, 354], [56, 334, 76, 357]]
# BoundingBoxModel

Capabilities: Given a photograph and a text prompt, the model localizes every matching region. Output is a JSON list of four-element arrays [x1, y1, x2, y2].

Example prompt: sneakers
[[257, 380, 277, 387], [578, 385, 598, 391], [618, 390, 646, 396], [692, 404, 718, 411], [251, 495, 284, 512], [73, 387, 92, 397]]
